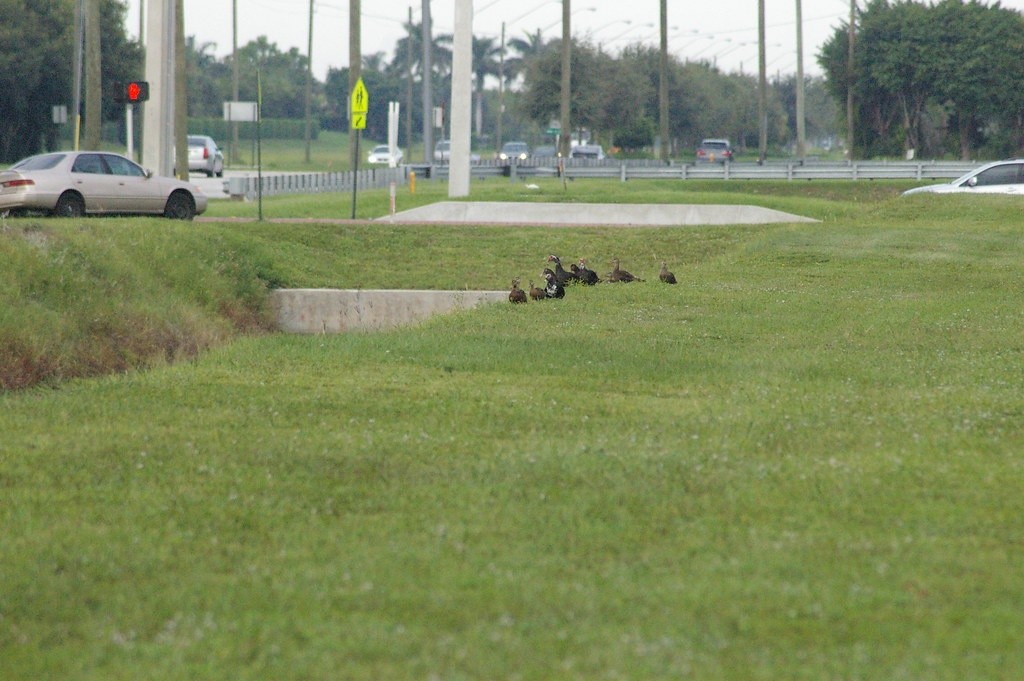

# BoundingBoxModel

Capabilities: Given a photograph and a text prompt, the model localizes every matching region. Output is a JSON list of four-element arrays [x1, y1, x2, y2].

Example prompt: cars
[[899, 159, 1024, 194], [0, 151, 209, 220], [173, 134, 224, 178], [499, 140, 531, 165], [569, 144, 605, 161], [534, 146, 558, 162], [367, 143, 403, 168]]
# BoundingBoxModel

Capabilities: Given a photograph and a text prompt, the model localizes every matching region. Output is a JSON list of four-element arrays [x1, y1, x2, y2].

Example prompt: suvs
[[694, 138, 735, 164]]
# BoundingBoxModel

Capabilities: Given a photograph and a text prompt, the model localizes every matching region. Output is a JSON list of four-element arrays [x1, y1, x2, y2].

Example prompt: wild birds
[[509, 277, 528, 304], [659, 259, 678, 284], [525, 184, 539, 190], [529, 254, 603, 300], [605, 257, 646, 283]]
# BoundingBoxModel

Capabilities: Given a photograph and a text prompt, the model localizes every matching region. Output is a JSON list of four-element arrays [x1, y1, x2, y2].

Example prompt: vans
[[435, 140, 448, 164]]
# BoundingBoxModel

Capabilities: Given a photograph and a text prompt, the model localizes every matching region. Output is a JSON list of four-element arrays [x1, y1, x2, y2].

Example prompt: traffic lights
[[116, 82, 150, 104]]
